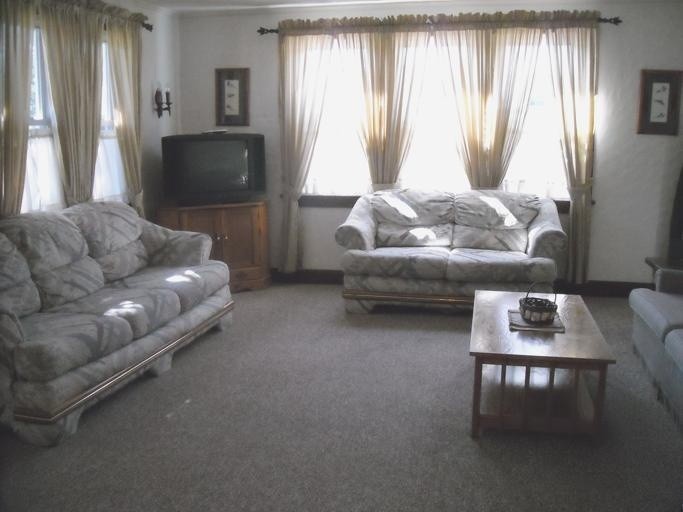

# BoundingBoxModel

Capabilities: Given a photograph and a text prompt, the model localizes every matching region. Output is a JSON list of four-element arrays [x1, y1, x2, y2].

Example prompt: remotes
[[202, 129, 229, 134]]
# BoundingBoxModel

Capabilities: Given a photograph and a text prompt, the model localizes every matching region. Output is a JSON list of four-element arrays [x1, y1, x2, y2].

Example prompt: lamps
[[153, 88, 171, 118]]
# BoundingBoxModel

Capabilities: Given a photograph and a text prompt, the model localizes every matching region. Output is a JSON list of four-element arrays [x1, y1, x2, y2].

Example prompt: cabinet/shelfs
[[157, 199, 272, 293]]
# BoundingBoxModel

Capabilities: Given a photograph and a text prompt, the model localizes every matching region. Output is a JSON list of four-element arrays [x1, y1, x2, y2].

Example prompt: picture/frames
[[636, 69, 683, 136], [214, 67, 250, 126]]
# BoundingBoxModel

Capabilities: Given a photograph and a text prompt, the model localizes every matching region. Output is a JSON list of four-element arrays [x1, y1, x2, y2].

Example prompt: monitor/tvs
[[160, 133, 266, 205]]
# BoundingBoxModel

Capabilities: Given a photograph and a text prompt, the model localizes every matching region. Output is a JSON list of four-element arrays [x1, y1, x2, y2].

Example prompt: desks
[[645, 257, 683, 290]]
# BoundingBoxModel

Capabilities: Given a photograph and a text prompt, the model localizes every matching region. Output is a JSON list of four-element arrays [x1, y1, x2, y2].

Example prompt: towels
[[508, 309, 565, 333]]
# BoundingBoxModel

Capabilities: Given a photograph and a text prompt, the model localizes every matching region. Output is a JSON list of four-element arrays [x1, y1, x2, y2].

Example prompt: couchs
[[628, 268, 683, 431], [0, 201, 234, 448], [335, 191, 570, 315]]
